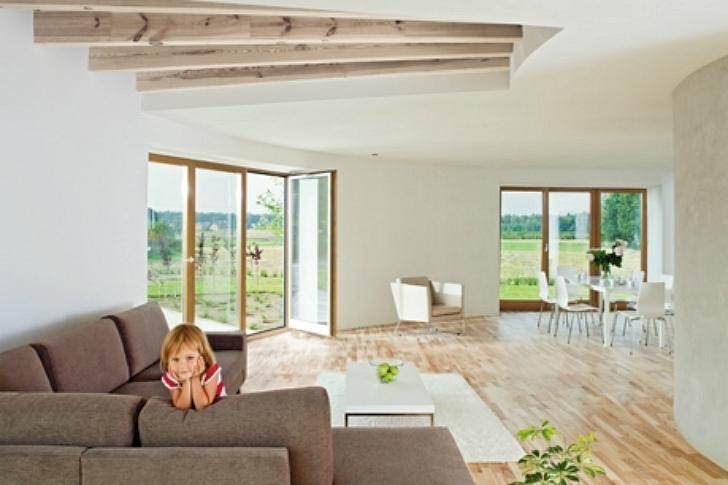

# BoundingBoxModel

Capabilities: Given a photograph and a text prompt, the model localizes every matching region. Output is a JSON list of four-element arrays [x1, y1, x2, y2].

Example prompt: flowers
[[586, 239, 625, 278]]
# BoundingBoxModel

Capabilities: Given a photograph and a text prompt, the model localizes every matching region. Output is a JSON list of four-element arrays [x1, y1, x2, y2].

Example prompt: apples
[[378, 363, 400, 383]]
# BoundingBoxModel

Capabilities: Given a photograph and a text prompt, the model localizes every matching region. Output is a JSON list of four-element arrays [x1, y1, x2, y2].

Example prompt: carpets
[[315, 371, 528, 465]]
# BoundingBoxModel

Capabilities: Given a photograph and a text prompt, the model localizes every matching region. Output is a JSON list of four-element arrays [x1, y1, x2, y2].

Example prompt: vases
[[601, 270, 613, 287]]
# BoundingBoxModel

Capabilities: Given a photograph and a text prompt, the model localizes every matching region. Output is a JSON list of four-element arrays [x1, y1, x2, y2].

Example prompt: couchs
[[0, 302, 247, 401], [0, 391, 479, 485]]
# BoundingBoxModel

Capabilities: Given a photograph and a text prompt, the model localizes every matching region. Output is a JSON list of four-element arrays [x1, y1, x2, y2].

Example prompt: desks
[[567, 274, 639, 335]]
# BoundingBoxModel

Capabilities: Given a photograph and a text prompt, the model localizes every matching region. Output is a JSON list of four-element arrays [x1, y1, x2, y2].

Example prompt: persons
[[606, 238, 626, 269], [157, 321, 227, 414]]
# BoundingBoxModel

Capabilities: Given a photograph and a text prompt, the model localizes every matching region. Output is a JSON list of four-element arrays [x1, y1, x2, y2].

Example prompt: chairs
[[617, 281, 672, 357], [391, 277, 465, 337], [535, 271, 571, 333], [553, 275, 604, 344]]
